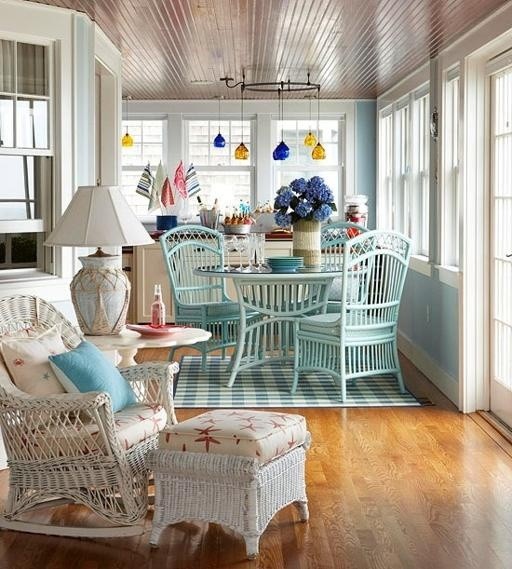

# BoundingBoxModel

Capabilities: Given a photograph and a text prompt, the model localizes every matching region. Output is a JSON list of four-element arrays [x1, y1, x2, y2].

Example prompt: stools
[[141, 406, 316, 562]]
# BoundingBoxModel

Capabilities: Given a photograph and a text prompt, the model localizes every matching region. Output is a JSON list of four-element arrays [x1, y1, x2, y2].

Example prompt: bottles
[[344, 194, 369, 228], [195, 196, 207, 210], [226, 211, 252, 224], [238, 199, 273, 213], [213, 199, 218, 210], [150, 283, 166, 328], [345, 206, 360, 236]]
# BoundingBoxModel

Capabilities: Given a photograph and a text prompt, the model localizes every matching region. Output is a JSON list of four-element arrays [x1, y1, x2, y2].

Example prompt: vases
[[291, 216, 323, 268]]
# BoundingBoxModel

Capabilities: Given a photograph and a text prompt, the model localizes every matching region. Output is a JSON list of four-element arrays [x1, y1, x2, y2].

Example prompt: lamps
[[211, 94, 227, 149], [120, 94, 136, 148], [39, 178, 159, 339], [215, 65, 329, 161]]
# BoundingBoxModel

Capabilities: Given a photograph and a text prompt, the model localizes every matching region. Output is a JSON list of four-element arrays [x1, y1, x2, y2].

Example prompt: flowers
[[272, 175, 338, 230]]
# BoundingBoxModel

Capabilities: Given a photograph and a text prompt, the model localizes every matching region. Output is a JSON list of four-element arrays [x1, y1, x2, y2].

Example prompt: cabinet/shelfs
[[134, 234, 176, 325], [265, 239, 309, 307], [179, 238, 260, 326], [320, 245, 363, 305]]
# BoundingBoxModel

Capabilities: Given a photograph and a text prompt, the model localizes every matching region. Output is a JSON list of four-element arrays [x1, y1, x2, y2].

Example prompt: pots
[[196, 211, 223, 228]]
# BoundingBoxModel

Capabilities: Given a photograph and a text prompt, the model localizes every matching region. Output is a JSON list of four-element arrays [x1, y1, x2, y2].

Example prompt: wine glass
[[180, 210, 193, 227]]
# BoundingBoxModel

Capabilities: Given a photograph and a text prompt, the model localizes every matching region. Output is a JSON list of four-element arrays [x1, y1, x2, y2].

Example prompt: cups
[[158, 216, 177, 230]]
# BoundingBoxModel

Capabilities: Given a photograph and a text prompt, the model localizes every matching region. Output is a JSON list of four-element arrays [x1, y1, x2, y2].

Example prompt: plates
[[266, 257, 304, 272], [125, 324, 185, 337]]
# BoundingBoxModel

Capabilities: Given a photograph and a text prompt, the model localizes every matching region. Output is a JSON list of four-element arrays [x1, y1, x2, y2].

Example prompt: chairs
[[281, 221, 376, 363], [288, 227, 410, 396], [157, 221, 266, 374], [1, 293, 185, 542]]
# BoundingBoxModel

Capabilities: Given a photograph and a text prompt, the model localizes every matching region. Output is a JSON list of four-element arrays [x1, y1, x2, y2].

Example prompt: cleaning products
[[245, 201, 251, 214], [232, 205, 239, 217], [225, 206, 230, 218], [238, 200, 244, 213]]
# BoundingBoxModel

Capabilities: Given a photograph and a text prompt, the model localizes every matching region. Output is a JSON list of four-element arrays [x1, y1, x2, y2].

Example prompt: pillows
[[0, 317, 74, 434], [45, 334, 139, 420]]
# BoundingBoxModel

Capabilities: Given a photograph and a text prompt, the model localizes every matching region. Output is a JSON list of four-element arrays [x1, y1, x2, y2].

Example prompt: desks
[[77, 320, 215, 404], [191, 260, 369, 390]]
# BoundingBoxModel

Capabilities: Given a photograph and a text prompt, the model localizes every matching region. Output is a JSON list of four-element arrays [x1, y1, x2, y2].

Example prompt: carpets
[[172, 349, 424, 409]]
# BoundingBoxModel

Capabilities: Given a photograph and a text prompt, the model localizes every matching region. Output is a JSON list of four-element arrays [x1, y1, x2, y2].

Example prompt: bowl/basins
[[222, 224, 253, 234]]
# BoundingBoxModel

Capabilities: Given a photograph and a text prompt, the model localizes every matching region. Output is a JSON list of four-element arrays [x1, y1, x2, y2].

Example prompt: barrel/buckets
[[199, 209, 220, 229]]
